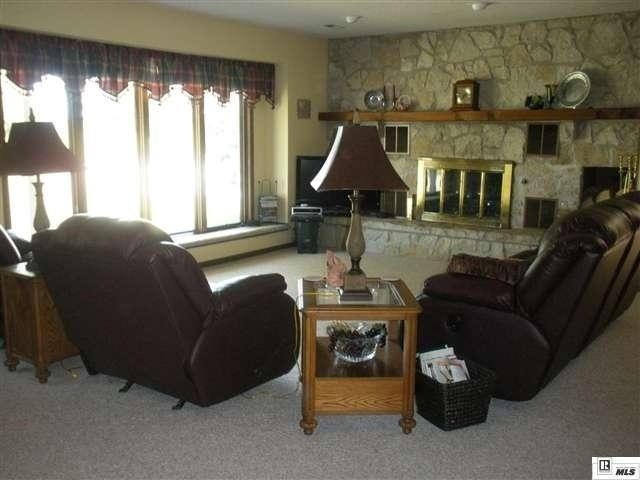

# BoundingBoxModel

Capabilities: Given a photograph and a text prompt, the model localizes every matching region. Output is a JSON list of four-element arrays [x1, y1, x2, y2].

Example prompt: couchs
[[398, 188, 639, 404]]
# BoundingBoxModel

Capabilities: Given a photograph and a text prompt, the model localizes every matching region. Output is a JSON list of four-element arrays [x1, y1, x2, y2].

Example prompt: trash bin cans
[[295, 214, 322, 253]]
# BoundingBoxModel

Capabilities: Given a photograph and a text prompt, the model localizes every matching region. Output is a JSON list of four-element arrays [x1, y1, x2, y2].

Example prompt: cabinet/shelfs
[[292, 274, 424, 435]]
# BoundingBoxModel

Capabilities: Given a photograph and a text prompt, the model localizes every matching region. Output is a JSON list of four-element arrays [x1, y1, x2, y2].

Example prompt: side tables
[[1, 258, 80, 384]]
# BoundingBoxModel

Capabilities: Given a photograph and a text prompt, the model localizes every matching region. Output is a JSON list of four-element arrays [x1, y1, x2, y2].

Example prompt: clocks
[[451, 77, 479, 111]]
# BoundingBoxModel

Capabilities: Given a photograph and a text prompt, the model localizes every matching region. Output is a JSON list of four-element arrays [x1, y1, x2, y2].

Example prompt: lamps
[[1, 106, 83, 273], [307, 125, 412, 276], [463, 0, 491, 11], [343, 13, 363, 25]]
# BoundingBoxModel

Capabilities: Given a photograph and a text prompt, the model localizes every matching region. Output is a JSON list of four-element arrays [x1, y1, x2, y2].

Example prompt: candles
[[546, 86, 551, 103], [383, 85, 387, 103], [392, 84, 395, 101]]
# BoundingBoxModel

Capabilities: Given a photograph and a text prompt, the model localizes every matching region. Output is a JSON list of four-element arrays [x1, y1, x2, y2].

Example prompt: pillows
[[444, 250, 525, 283]]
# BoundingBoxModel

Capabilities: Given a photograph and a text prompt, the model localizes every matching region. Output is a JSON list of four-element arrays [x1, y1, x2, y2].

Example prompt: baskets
[[415, 355, 498, 432]]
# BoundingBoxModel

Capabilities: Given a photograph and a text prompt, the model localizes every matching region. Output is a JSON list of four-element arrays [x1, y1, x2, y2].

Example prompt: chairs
[[28, 212, 300, 414], [1, 220, 32, 266]]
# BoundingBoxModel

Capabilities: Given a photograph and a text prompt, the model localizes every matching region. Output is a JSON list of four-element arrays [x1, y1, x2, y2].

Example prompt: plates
[[558, 70, 594, 107]]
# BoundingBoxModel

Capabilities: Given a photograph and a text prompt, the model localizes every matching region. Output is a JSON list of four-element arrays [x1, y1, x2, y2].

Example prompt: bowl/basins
[[328, 321, 384, 363]]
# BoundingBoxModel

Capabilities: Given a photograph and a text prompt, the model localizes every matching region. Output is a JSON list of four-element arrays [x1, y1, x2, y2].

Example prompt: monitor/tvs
[[295, 155, 382, 219]]
